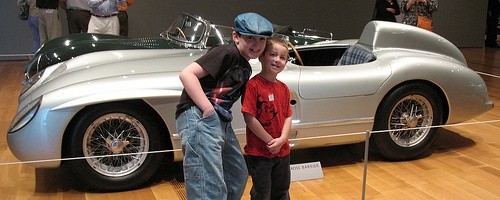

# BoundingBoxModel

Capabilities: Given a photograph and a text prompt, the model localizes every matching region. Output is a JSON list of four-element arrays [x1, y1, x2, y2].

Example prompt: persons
[[86, 0, 120, 36], [116, 0, 134, 36], [176, 12, 274, 200], [241, 38, 293, 200], [374, 0, 400, 22], [36, 0, 69, 47], [399, 0, 439, 31], [17, 0, 40, 54], [66, 0, 91, 34]]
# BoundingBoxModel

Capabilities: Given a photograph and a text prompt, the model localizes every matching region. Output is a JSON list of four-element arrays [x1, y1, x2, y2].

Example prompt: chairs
[[336, 44, 375, 67]]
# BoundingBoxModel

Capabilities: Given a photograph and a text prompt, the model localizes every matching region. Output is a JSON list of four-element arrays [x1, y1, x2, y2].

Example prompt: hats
[[234, 13, 273, 38]]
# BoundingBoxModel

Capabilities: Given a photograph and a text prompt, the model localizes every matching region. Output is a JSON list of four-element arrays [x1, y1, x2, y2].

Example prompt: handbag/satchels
[[417, 16, 432, 31], [18, 2, 30, 21]]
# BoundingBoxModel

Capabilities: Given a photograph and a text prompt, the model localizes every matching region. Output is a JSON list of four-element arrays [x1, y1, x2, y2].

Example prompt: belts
[[39, 7, 58, 10], [92, 13, 116, 18]]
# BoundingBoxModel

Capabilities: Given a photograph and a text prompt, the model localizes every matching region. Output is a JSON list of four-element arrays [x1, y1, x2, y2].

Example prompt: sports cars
[[6, 11, 495, 192]]
